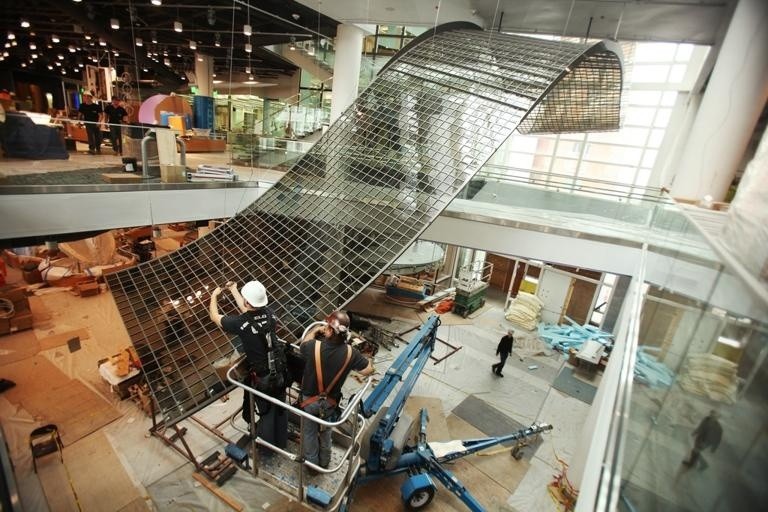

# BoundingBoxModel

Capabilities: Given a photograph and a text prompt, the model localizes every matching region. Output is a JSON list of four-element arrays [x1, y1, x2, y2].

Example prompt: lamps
[[0, 0, 327, 80]]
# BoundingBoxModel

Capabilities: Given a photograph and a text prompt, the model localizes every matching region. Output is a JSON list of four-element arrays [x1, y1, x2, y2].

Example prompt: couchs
[[5, 111, 69, 160]]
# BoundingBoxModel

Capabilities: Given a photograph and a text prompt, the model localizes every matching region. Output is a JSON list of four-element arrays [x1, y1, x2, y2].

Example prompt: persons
[[299, 311, 376, 474], [682, 409, 722, 466], [78, 95, 128, 156], [210, 280, 289, 457], [492, 329, 514, 378]]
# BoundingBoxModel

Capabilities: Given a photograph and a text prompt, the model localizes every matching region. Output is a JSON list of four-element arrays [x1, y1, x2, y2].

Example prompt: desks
[[96, 352, 142, 400], [574, 339, 606, 375]]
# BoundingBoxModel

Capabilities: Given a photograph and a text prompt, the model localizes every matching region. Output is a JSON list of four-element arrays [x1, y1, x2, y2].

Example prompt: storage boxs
[[161, 164, 186, 183]]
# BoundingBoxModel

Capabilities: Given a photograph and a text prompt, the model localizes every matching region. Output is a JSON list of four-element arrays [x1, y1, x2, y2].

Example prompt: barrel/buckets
[[194, 95, 214, 132], [122, 157, 138, 172], [46, 241, 58, 251], [153, 229, 161, 238]]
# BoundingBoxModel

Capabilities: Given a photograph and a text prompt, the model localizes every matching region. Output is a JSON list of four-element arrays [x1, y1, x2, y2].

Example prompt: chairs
[[27, 423, 63, 474]]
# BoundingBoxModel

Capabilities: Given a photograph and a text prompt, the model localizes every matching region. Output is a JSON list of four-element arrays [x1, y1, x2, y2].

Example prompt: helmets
[[508, 328, 515, 334], [241, 281, 268, 308], [83, 90, 92, 97], [326, 310, 351, 335]]
